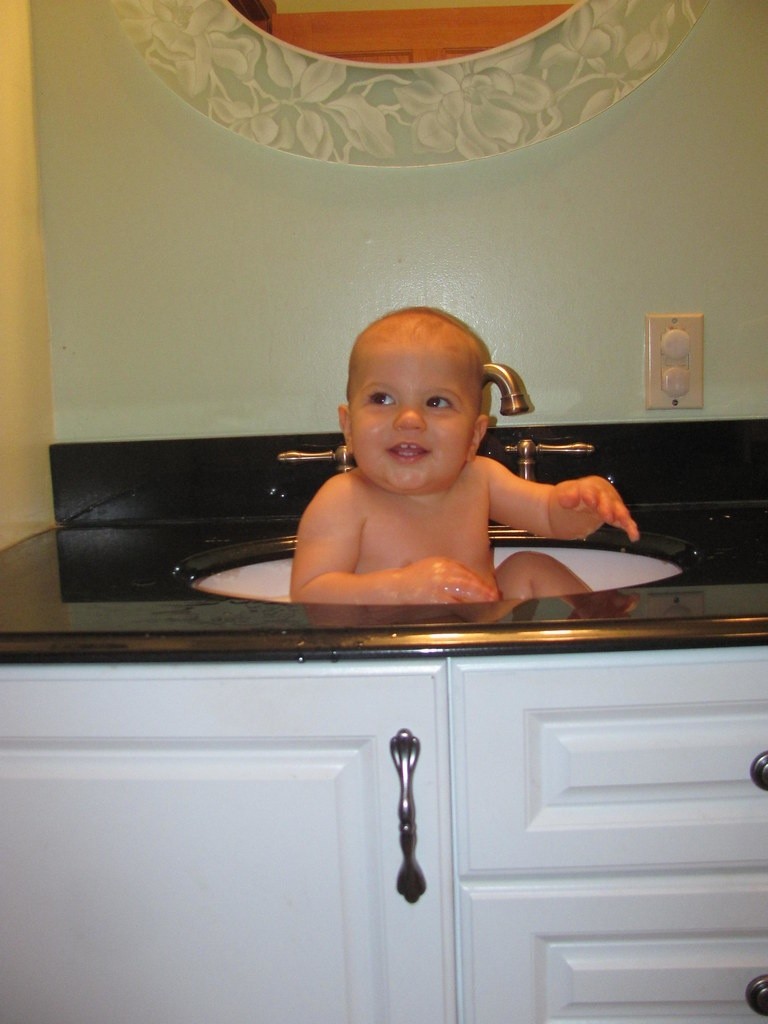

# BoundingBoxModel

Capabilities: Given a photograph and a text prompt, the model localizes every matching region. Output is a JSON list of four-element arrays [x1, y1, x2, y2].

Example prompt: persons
[[291, 307, 640, 605]]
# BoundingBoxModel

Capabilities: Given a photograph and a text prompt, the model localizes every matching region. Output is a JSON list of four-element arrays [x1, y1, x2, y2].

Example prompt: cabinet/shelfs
[[1, 659, 458, 1022], [450, 649, 768, 1024]]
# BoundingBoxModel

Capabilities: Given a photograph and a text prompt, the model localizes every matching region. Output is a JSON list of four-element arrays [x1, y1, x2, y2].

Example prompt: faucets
[[481, 364, 532, 419]]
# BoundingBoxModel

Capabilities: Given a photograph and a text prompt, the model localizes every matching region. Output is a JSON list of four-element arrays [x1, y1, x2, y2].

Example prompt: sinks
[[190, 521, 685, 607]]
